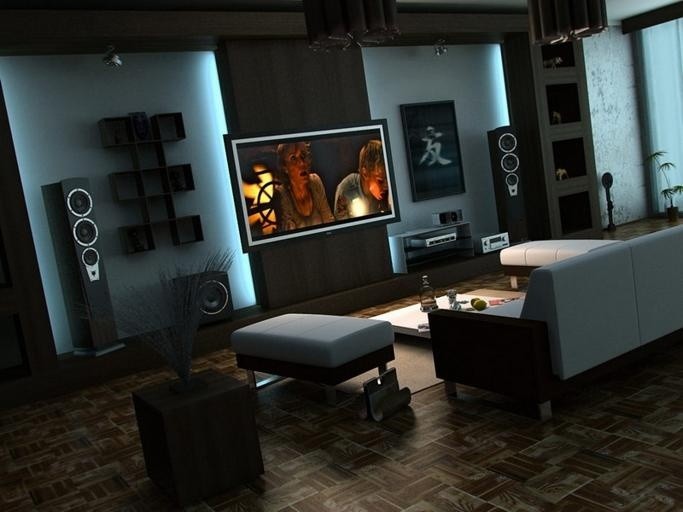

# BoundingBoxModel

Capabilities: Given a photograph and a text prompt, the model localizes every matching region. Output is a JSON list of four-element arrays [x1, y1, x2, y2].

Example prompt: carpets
[[334, 289, 527, 395]]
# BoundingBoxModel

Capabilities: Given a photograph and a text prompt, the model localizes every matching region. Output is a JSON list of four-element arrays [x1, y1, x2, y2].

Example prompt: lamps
[[434, 39, 448, 56], [103, 52, 121, 67]]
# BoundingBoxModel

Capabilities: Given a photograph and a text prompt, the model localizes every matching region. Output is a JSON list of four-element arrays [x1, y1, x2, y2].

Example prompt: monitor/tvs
[[222, 118, 401, 253]]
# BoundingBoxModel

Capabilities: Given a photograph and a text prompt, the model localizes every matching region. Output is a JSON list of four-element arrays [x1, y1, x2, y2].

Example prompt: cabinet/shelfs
[[532, 40, 602, 240], [389, 222, 475, 274], [97, 111, 204, 255]]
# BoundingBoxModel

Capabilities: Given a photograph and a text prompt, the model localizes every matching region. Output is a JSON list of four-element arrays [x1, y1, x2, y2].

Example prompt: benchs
[[500, 239, 624, 289], [230, 314, 395, 406]]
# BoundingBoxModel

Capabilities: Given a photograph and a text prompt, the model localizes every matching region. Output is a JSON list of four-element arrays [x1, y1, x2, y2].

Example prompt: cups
[[446, 289, 458, 306]]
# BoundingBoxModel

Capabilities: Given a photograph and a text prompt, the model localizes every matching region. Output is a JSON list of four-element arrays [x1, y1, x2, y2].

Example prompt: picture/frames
[[399, 100, 466, 202]]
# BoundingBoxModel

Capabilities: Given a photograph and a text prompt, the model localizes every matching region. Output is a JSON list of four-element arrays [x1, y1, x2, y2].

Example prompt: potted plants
[[646, 152, 683, 222]]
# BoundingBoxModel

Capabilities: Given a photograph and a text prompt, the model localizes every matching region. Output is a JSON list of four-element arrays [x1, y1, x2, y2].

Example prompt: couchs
[[427, 225, 683, 421]]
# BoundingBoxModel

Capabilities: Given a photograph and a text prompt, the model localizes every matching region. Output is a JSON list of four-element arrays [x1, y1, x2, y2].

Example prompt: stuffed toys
[[446, 287, 462, 311]]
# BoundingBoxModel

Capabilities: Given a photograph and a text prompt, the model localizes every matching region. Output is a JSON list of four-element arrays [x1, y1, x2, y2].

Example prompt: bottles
[[418, 275, 439, 312]]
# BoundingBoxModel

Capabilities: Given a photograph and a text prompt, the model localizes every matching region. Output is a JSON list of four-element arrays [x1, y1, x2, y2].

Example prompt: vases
[[170, 362, 204, 392]]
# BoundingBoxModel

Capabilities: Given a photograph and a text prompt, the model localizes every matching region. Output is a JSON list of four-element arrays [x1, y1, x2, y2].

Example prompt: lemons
[[473, 300, 487, 310]]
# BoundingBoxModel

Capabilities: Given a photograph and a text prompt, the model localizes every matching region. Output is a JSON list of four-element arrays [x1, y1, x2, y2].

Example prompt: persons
[[332, 139, 387, 221], [269, 141, 335, 234]]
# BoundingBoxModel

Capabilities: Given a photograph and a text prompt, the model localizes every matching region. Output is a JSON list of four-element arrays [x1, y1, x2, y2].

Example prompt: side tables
[[132, 369, 265, 509]]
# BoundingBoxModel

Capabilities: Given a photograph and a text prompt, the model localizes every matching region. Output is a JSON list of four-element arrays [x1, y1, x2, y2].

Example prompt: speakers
[[41, 175, 126, 356], [486, 125, 552, 246], [171, 271, 234, 326]]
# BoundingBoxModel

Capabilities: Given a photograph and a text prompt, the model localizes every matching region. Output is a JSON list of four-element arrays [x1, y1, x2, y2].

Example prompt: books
[[487, 297, 519, 307], [418, 323, 430, 334]]
